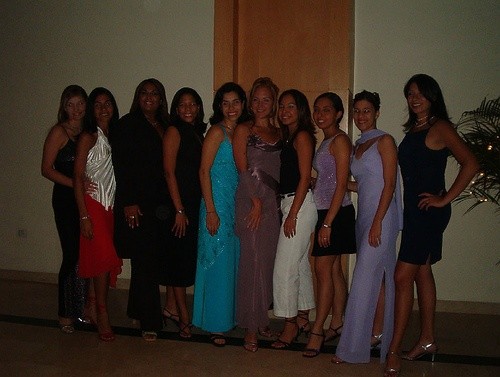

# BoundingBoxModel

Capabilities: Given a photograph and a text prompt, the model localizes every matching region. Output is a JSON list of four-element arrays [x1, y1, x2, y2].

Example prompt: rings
[[129, 216, 135, 219]]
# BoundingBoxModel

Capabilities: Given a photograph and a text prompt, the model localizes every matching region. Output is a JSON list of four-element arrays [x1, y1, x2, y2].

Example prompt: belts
[[280, 188, 309, 199]]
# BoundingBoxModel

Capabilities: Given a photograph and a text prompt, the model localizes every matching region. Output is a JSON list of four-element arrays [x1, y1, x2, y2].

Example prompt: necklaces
[[415, 113, 436, 128], [147, 118, 160, 129]]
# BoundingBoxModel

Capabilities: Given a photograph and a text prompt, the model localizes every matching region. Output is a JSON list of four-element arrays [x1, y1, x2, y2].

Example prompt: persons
[[302, 92, 357, 358], [270, 89, 318, 350], [113, 78, 170, 342], [330, 90, 404, 364], [42, 84, 98, 333], [74, 88, 120, 342], [384, 73, 480, 377], [163, 88, 208, 338], [192, 83, 251, 347], [233, 77, 284, 352]]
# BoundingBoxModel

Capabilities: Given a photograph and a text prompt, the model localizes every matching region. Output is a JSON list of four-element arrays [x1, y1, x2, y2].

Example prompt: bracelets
[[322, 224, 332, 228], [207, 211, 216, 215], [176, 209, 186, 214], [289, 216, 298, 220], [80, 216, 91, 221]]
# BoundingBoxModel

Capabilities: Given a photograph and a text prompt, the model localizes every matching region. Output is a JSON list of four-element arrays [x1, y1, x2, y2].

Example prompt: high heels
[[84, 300, 115, 340], [402, 340, 439, 362], [303, 327, 325, 357], [384, 350, 401, 377], [332, 333, 384, 365], [272, 311, 311, 349], [324, 323, 343, 342]]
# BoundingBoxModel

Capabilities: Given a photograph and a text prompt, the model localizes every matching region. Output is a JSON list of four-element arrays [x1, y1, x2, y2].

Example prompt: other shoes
[[141, 330, 157, 342], [161, 305, 192, 338], [57, 320, 74, 334], [243, 326, 273, 352], [214, 335, 225, 347]]
